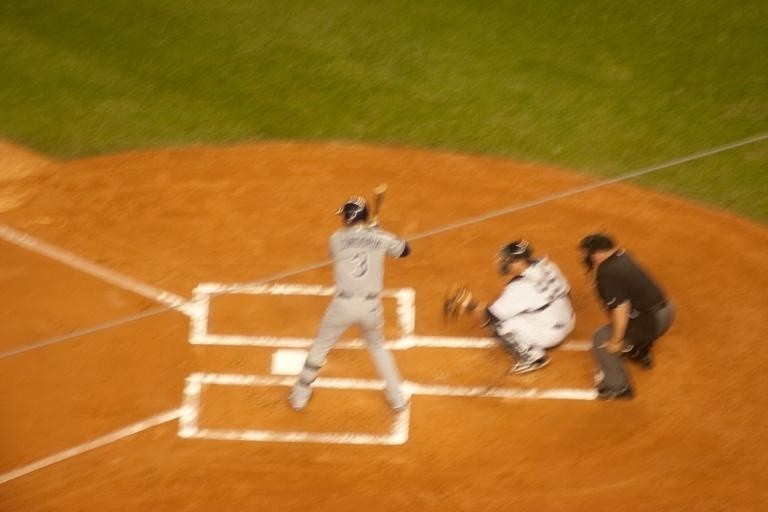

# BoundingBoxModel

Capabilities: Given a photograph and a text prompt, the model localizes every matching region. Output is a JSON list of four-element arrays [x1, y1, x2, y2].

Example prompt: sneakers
[[289, 383, 313, 410], [630, 348, 652, 366], [512, 347, 551, 375], [595, 381, 630, 399]]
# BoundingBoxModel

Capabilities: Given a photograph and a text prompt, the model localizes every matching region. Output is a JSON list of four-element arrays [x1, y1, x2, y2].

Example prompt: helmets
[[496, 241, 531, 275], [577, 235, 612, 275], [335, 196, 368, 226]]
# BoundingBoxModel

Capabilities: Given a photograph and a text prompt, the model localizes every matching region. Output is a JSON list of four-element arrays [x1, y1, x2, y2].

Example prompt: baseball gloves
[[443, 284, 479, 319]]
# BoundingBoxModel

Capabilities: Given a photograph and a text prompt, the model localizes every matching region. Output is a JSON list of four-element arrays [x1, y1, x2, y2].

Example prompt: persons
[[290, 197, 412, 412], [578, 234, 675, 399], [473, 238, 576, 375]]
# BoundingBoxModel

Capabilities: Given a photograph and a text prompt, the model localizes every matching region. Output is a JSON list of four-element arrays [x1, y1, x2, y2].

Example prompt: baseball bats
[[372, 182, 387, 223]]
